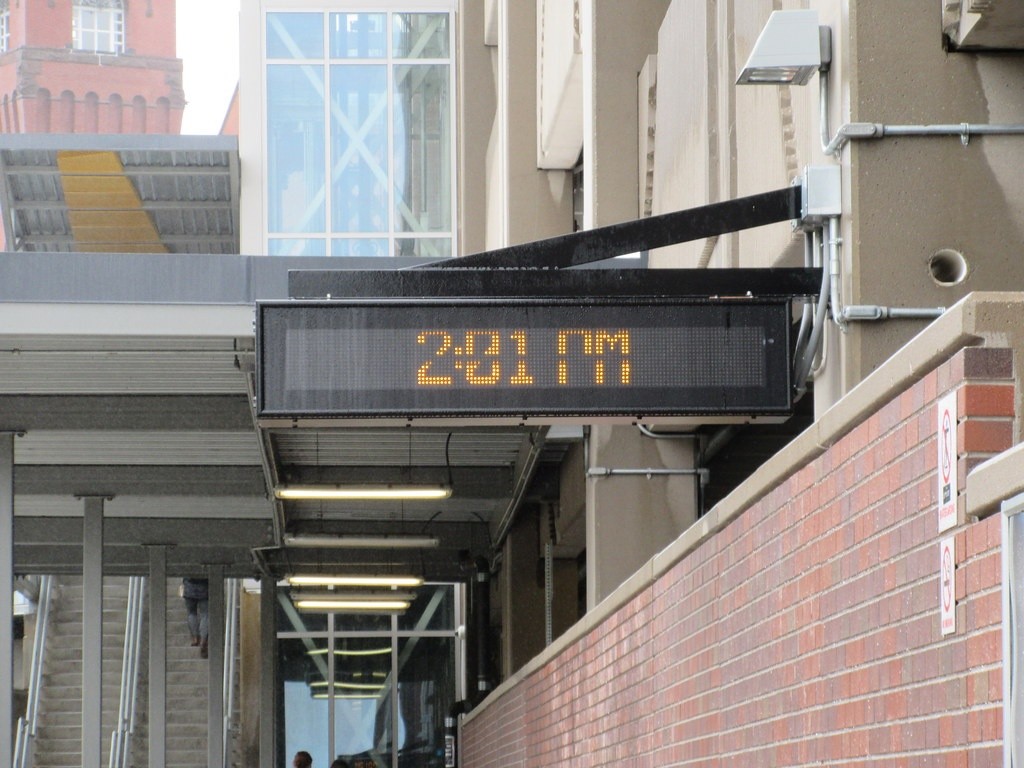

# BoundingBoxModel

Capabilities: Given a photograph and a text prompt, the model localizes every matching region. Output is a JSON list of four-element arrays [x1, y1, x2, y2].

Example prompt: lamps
[[293, 599, 410, 610], [283, 549, 425, 587], [298, 608, 407, 615], [290, 591, 418, 601], [282, 509, 439, 549], [273, 434, 456, 500], [735, 10, 838, 86]]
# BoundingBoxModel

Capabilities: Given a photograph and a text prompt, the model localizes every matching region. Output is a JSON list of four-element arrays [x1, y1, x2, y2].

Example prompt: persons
[[330, 760, 347, 768], [293, 750, 312, 768], [182, 578, 208, 660]]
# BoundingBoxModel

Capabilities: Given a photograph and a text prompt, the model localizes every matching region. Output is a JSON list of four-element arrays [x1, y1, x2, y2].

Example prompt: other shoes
[[200, 640, 208, 659], [191, 636, 199, 646]]
